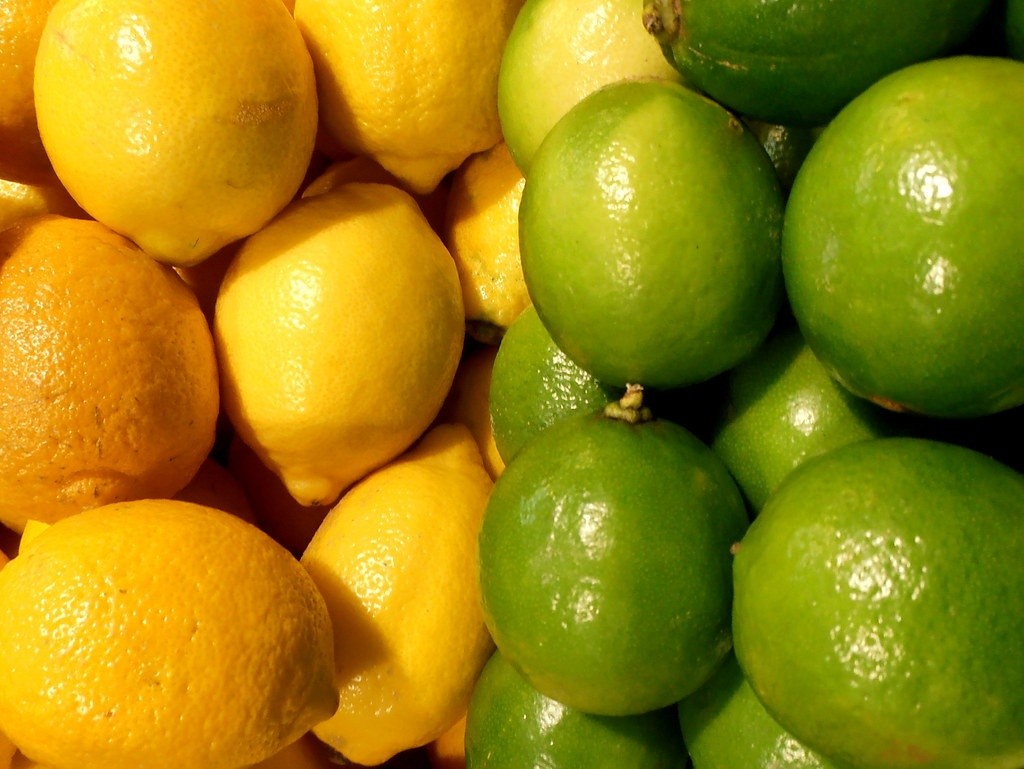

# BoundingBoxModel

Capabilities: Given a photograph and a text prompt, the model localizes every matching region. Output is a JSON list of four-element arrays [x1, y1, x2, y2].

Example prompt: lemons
[[0, 2, 1024, 769]]
